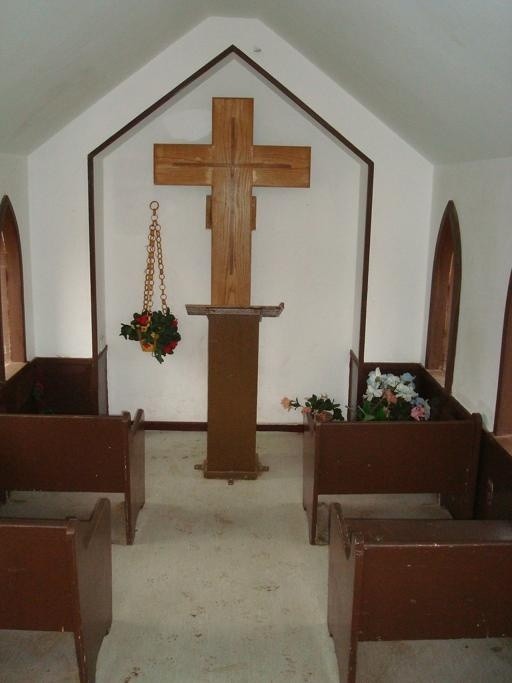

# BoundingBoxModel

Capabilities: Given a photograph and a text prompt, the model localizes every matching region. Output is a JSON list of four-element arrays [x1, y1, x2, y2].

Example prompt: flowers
[[118, 310, 182, 364], [353, 366, 432, 420], [278, 392, 346, 422]]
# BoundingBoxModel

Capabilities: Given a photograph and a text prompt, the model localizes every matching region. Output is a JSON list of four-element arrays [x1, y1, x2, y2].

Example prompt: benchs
[[302, 411, 482, 545], [1, 408, 145, 547], [0, 498, 112, 682], [327, 501, 509, 683]]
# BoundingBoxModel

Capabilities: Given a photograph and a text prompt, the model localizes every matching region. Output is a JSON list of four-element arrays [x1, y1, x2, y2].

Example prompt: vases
[[140, 338, 155, 352]]
